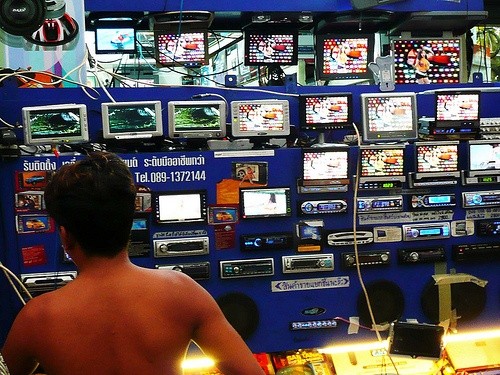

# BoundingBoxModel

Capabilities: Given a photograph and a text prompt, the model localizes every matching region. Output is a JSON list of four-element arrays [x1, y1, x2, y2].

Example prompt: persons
[[1, 151, 264, 375], [413, 46, 434, 84], [111, 33, 131, 50], [338, 43, 351, 69], [263, 38, 276, 62], [173, 40, 190, 61], [237, 167, 256, 185]]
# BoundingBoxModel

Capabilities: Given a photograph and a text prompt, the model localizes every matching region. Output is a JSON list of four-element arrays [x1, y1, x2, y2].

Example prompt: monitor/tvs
[[94, 18, 468, 83], [21, 90, 500, 225]]
